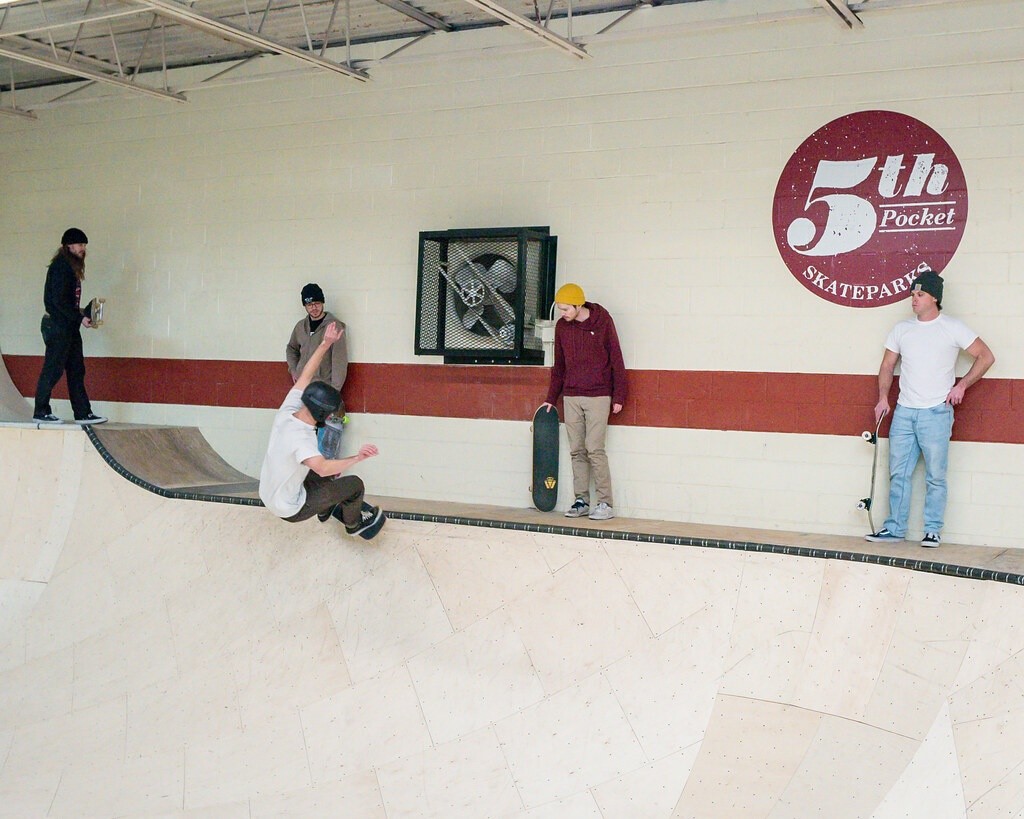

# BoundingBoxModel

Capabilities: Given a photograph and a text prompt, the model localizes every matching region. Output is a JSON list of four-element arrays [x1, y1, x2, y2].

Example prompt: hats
[[911, 271, 944, 303], [555, 283, 585, 305], [301, 283, 325, 306], [61, 228, 88, 245]]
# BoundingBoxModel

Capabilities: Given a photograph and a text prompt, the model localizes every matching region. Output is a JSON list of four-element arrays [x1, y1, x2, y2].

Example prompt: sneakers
[[344, 506, 382, 536], [565, 501, 590, 517], [74, 413, 108, 425], [865, 527, 906, 542], [33, 414, 62, 424], [589, 502, 615, 520], [920, 532, 941, 547], [318, 499, 341, 523]]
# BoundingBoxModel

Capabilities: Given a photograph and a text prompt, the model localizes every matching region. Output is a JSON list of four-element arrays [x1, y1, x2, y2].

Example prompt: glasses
[[305, 301, 321, 309]]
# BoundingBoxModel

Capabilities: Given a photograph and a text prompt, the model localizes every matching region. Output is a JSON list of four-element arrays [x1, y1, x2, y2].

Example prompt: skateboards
[[315, 397, 352, 481], [327, 490, 387, 541], [528, 405, 559, 513], [856, 409, 887, 536], [83, 297, 106, 328]]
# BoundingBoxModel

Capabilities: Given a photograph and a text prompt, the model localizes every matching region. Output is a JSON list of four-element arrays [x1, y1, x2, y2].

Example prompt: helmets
[[302, 380, 341, 423]]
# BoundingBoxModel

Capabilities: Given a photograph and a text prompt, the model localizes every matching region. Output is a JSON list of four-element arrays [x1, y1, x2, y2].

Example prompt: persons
[[32, 228, 108, 424], [258, 323, 383, 537], [540, 283, 625, 519], [865, 270, 994, 548], [286, 284, 348, 481]]
